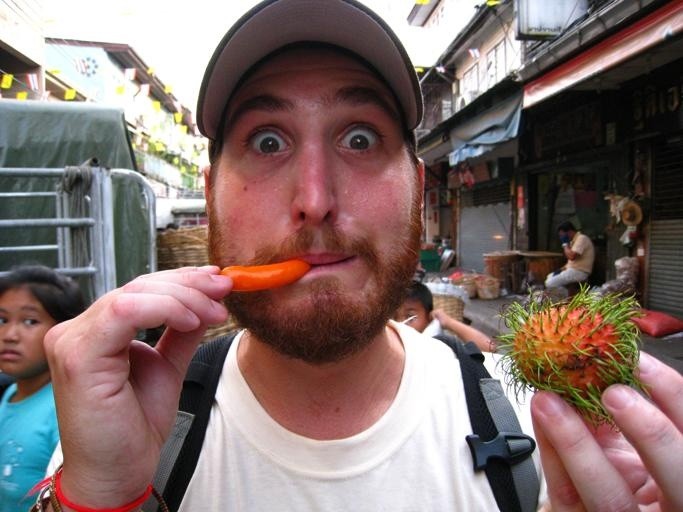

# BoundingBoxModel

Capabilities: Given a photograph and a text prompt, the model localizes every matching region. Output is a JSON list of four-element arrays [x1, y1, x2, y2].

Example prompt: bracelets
[[30, 469, 169, 512]]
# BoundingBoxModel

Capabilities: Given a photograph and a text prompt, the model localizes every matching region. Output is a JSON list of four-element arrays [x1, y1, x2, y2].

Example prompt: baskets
[[450, 272, 501, 300], [156, 224, 239, 343], [433, 293, 465, 323]]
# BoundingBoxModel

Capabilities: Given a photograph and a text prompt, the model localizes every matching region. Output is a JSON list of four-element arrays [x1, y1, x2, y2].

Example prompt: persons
[[543, 220, 596, 297], [0, 265, 86, 511], [30, 0, 683, 512], [392, 282, 499, 351]]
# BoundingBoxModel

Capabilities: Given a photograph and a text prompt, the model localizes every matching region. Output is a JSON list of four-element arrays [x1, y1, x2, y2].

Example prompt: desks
[[516, 250, 564, 284]]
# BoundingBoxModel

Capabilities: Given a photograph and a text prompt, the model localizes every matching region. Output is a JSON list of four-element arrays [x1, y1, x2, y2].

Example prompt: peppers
[[218, 258, 312, 293]]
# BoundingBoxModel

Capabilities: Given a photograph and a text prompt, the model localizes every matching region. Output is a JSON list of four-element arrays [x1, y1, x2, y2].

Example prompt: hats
[[196, 0, 425, 141]]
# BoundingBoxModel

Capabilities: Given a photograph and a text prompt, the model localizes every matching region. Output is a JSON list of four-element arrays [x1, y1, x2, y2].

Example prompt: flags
[[1, 67, 205, 175]]
